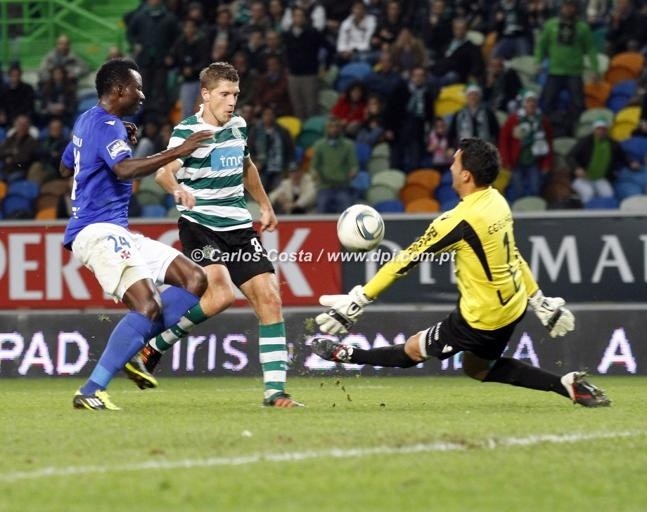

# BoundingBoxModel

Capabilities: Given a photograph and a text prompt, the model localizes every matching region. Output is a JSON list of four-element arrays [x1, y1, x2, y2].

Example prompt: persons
[[124, 62, 303, 409], [311, 138, 611, 405], [60, 60, 213, 412], [2, 1, 647, 223]]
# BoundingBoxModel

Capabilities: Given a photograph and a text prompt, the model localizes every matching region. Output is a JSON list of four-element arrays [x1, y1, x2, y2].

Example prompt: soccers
[[335, 205, 385, 253]]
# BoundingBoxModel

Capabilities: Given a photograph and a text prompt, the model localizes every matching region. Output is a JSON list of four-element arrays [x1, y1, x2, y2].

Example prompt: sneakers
[[560, 370, 614, 409], [265, 394, 304, 408], [72, 389, 121, 411], [125, 355, 159, 390], [310, 337, 359, 364]]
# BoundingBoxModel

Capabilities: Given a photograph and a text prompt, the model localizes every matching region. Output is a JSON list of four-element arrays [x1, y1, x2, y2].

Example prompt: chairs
[[1, 50, 646, 221]]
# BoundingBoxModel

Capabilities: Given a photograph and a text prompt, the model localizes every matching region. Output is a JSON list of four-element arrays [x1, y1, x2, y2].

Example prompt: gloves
[[314, 283, 377, 337], [528, 289, 575, 339]]
